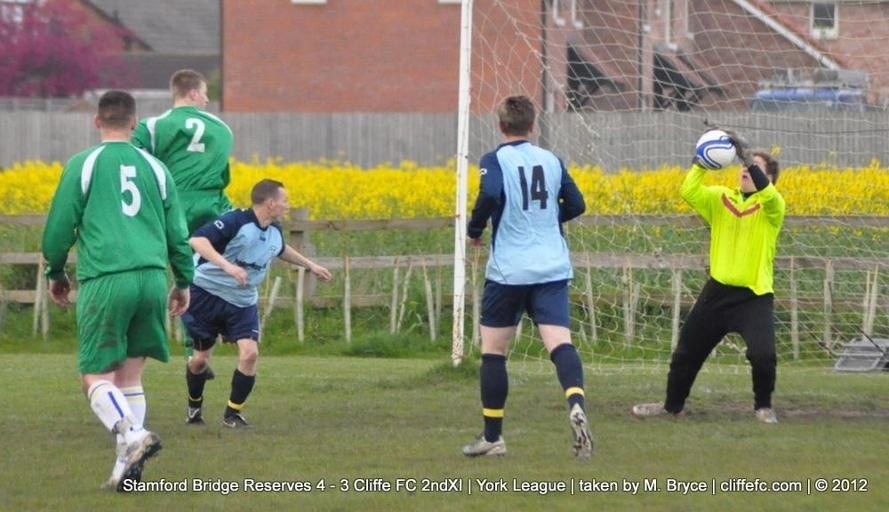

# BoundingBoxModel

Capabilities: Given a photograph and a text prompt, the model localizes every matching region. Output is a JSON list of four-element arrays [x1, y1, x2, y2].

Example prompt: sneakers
[[221, 413, 249, 428], [755, 407, 778, 424], [185, 407, 205, 424], [462, 433, 507, 456], [569, 403, 594, 460], [631, 401, 667, 418], [100, 416, 163, 492]]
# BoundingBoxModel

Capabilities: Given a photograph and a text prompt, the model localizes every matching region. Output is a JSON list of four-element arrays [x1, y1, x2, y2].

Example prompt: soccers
[[695, 129, 738, 170]]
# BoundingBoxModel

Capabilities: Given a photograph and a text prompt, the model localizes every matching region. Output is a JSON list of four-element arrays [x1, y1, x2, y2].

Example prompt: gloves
[[729, 130, 755, 167]]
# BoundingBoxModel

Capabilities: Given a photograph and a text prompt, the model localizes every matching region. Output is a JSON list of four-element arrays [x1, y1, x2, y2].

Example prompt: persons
[[41, 91, 197, 493], [462, 94, 594, 459], [131, 69, 235, 236], [185, 176, 332, 430], [632, 128, 786, 424]]
